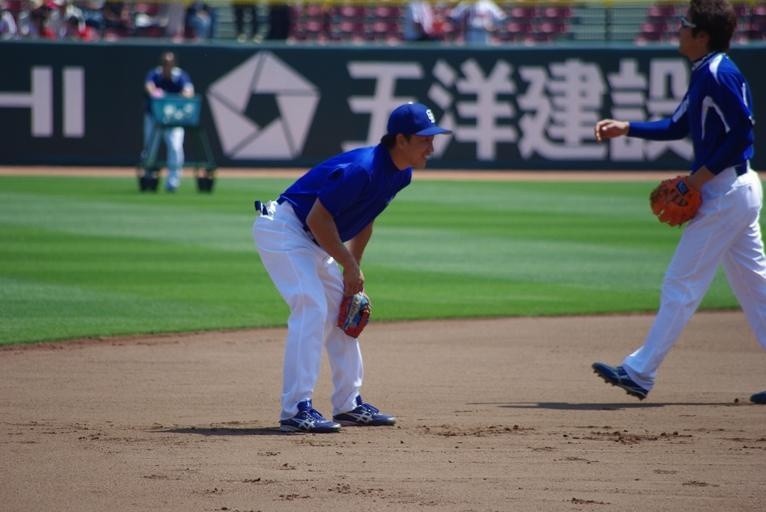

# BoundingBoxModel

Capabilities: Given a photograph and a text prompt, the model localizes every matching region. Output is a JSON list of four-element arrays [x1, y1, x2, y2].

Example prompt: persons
[[253, 99, 453, 432], [142, 53, 194, 191], [591, 0, 766, 406], [405, 1, 509, 43], [0, 0, 293, 42]]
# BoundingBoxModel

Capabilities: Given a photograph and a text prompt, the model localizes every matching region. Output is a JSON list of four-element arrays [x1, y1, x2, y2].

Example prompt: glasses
[[680, 17, 697, 30]]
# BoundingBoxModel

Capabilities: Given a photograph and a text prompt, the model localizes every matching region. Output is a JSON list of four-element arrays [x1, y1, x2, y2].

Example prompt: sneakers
[[592, 361, 649, 400], [750, 390, 765, 404], [279, 399, 342, 433], [333, 395, 397, 426]]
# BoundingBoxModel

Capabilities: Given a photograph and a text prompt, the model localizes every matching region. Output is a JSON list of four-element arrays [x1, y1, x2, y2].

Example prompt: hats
[[386, 102, 453, 138]]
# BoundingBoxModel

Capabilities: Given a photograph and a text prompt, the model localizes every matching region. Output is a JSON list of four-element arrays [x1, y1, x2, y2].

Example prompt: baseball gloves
[[650, 175, 702, 226], [337, 291, 370, 338]]
